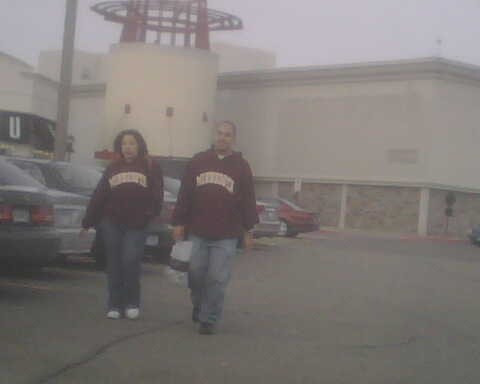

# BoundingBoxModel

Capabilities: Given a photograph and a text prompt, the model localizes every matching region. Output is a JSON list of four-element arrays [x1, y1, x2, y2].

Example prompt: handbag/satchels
[[164, 241, 193, 287]]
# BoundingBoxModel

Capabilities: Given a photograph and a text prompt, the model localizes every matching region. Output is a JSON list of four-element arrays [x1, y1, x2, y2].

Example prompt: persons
[[78, 129, 164, 320], [172, 120, 260, 335]]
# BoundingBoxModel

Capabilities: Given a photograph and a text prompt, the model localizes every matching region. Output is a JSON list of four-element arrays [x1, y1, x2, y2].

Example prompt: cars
[[0, 157, 97, 257], [5, 158, 178, 253], [272, 194, 320, 235], [163, 175, 279, 237]]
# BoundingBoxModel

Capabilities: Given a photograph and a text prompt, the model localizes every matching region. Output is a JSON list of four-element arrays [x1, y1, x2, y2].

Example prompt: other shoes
[[192, 307, 218, 335], [106, 311, 121, 320], [125, 308, 140, 319]]
